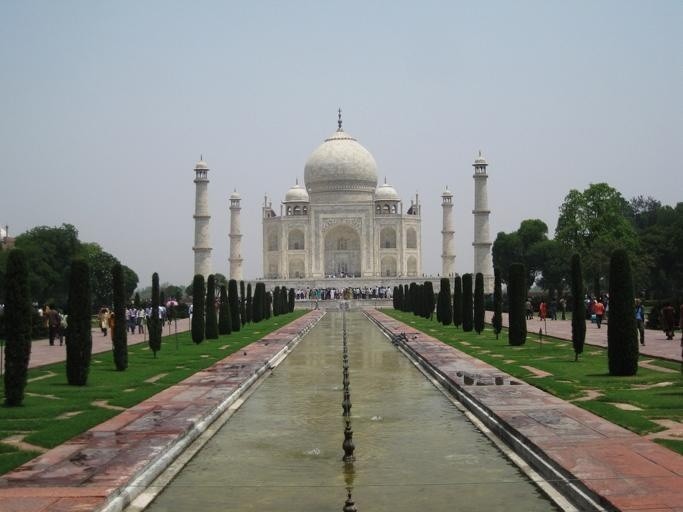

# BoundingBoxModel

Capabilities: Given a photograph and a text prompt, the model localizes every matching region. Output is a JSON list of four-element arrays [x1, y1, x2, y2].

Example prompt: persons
[[30, 299, 221, 346], [296, 286, 391, 299], [526, 297, 683, 357]]
